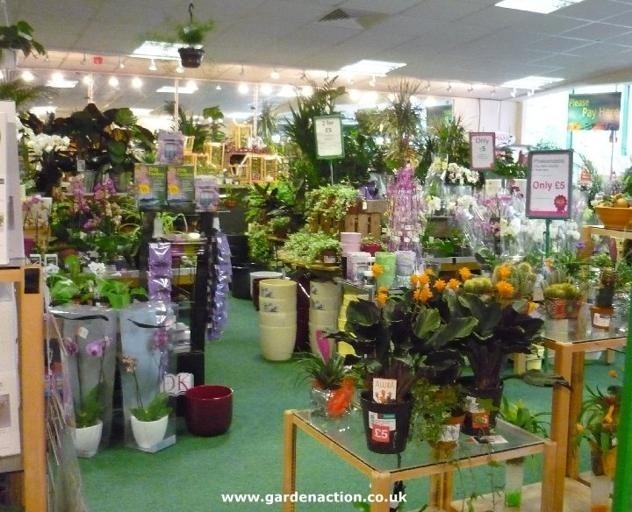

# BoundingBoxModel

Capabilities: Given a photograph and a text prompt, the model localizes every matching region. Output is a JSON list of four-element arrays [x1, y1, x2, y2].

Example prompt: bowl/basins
[[591, 205, 632, 231], [258, 278, 298, 361], [307, 279, 343, 358], [376, 251, 420, 289]]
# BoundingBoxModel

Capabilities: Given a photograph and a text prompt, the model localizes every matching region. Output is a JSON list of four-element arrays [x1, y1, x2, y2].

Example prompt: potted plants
[[137, 10, 215, 68], [0, 84, 632, 512]]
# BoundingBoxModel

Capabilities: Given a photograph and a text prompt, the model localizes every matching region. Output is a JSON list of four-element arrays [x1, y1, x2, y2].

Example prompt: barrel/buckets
[[341, 230, 362, 258], [260, 278, 297, 298], [260, 315, 297, 327], [259, 299, 297, 313], [307, 280, 369, 358], [346, 251, 416, 289], [260, 327, 296, 362]]
